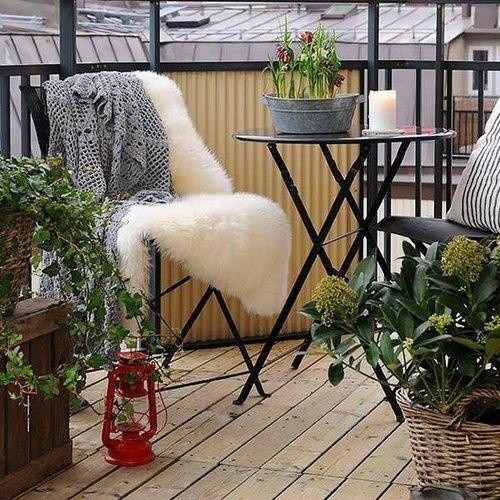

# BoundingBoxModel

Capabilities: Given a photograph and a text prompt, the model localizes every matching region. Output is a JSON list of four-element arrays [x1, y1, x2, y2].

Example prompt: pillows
[[446, 96, 500, 234]]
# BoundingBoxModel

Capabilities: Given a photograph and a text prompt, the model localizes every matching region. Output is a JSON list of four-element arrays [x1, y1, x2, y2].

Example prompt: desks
[[231, 126, 458, 424]]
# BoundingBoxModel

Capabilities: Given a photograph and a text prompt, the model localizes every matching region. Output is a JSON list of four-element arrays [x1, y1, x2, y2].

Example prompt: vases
[[259, 92, 366, 134]]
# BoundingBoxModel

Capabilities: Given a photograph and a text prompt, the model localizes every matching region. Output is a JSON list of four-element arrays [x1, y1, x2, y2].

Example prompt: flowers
[[262, 12, 345, 99]]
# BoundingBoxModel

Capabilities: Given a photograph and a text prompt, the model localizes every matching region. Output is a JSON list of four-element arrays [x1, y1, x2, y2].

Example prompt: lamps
[[101, 349, 158, 467]]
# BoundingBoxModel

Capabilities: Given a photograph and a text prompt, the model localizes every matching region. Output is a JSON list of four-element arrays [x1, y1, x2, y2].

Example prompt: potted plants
[[0, 155, 183, 425], [297, 234, 500, 500]]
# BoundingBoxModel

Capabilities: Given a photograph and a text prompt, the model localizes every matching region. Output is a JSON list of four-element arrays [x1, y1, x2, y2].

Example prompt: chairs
[[18, 83, 272, 401], [371, 214, 500, 402]]
